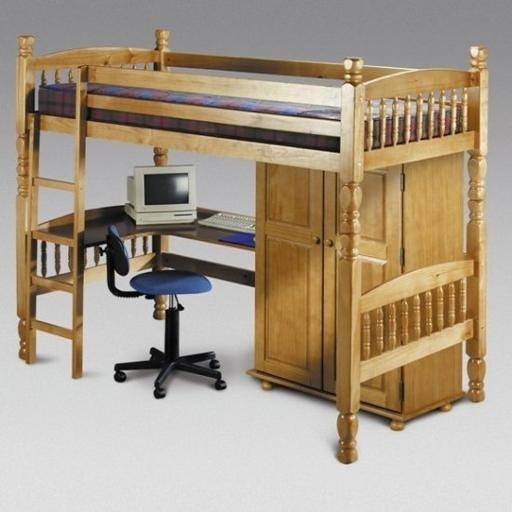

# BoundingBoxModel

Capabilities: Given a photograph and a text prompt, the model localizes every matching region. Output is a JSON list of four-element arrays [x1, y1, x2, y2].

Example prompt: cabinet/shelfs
[[247, 150, 467, 432]]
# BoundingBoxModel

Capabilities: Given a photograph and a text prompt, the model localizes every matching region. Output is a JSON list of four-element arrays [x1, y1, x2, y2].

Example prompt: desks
[[27, 204, 256, 363]]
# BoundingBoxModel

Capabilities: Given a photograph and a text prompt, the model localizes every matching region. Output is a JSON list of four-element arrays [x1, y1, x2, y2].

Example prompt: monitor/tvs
[[128, 163, 198, 211]]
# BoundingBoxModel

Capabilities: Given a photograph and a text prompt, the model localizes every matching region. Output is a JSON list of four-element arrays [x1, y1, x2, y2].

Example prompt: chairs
[[96, 226, 227, 400]]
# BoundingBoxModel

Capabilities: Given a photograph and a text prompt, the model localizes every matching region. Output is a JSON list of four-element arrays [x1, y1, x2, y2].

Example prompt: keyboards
[[198, 212, 256, 234]]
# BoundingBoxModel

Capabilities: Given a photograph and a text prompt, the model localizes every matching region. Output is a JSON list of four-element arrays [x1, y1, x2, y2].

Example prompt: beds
[[14, 28, 489, 465]]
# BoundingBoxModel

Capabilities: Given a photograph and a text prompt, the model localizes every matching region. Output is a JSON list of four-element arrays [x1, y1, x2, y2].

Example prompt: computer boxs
[[124, 203, 197, 225]]
